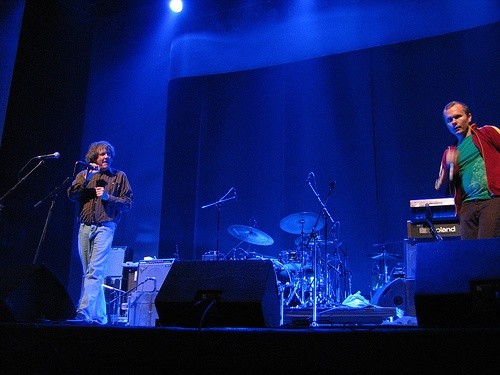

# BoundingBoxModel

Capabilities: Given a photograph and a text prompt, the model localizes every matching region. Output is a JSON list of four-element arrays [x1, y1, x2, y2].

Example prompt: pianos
[[405, 198, 465, 240]]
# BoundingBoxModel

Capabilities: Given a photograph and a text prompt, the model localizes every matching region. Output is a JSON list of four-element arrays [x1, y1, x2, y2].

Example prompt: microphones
[[253, 218, 257, 224], [79, 161, 98, 171], [37, 152, 60, 159], [449, 162, 454, 181], [146, 277, 156, 280]]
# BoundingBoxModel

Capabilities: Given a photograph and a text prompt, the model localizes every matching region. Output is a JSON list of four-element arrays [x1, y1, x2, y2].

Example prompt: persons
[[67, 141, 133, 325], [435, 101, 500, 240]]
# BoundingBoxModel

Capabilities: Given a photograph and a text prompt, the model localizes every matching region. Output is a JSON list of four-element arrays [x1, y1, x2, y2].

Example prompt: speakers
[[413, 238, 500, 328], [136, 258, 174, 291], [371, 277, 407, 316], [105, 245, 132, 277], [0, 265, 78, 322], [154, 260, 281, 329], [128, 292, 159, 327]]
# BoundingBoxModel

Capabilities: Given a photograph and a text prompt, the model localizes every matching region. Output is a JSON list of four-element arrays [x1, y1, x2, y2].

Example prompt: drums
[[247, 255, 316, 308]]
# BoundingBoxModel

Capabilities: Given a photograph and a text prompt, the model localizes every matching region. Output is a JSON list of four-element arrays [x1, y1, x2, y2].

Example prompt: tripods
[[275, 182, 349, 307]]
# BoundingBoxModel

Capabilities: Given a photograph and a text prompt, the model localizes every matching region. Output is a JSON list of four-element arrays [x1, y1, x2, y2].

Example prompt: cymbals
[[280, 211, 326, 235], [294, 235, 334, 246], [369, 252, 402, 260], [226, 224, 275, 245]]
